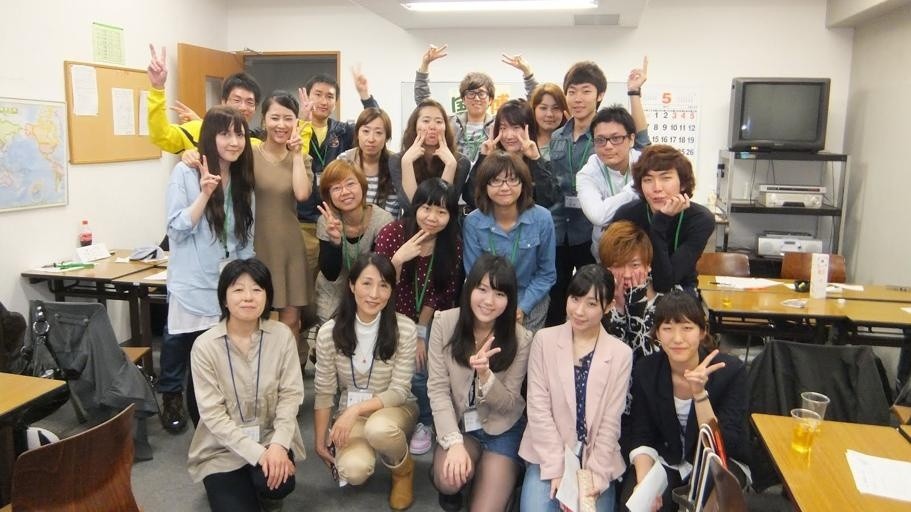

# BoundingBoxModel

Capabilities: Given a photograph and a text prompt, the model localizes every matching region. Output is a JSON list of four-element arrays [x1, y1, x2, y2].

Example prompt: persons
[[313, 251, 420, 510], [146, 43, 720, 456], [186, 258, 306, 512], [425, 255, 534, 511], [621, 289, 752, 511], [518, 264, 634, 510]]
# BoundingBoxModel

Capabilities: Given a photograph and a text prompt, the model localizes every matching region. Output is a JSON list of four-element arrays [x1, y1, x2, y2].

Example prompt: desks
[[747, 409, 911, 511], [696, 275, 911, 383], [21, 245, 168, 346], [0, 371, 71, 457]]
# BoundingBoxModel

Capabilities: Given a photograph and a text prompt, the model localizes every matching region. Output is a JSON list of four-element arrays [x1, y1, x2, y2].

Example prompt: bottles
[[78, 219, 93, 246]]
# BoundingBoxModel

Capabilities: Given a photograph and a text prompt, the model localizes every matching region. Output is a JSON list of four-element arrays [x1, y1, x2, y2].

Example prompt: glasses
[[464, 90, 489, 100], [592, 133, 631, 147], [487, 175, 522, 187]]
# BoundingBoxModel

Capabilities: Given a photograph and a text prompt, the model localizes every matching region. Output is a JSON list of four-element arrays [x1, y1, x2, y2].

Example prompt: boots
[[378, 442, 414, 511], [430, 474, 467, 512], [298, 328, 310, 376]]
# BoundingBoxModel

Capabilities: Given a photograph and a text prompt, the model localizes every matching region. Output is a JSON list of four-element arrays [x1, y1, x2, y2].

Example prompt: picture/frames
[[0, 95, 70, 213]]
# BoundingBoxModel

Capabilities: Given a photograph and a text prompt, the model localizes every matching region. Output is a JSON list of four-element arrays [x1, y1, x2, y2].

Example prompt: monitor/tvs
[[728, 78, 831, 151]]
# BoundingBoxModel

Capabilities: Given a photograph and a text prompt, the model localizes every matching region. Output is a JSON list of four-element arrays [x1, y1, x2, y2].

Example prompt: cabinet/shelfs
[[722, 148, 851, 276]]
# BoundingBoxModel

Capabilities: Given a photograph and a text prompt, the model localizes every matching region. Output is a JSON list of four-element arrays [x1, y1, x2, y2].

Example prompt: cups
[[789, 407, 821, 454], [801, 390, 832, 423], [810, 252, 830, 300]]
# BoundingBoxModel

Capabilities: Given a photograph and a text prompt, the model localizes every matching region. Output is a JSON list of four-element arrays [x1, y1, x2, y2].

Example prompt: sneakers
[[161, 390, 189, 434], [409, 423, 431, 455]]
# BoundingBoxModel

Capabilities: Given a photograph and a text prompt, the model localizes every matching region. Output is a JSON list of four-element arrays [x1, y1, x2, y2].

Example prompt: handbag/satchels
[[26, 299, 72, 380]]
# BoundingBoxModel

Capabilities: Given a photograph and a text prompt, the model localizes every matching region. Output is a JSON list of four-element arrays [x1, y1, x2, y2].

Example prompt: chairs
[[781, 251, 848, 281], [697, 420, 768, 512], [695, 253, 751, 276], [745, 339, 895, 424], [0, 303, 30, 373], [29, 296, 153, 417], [1, 401, 143, 511]]
